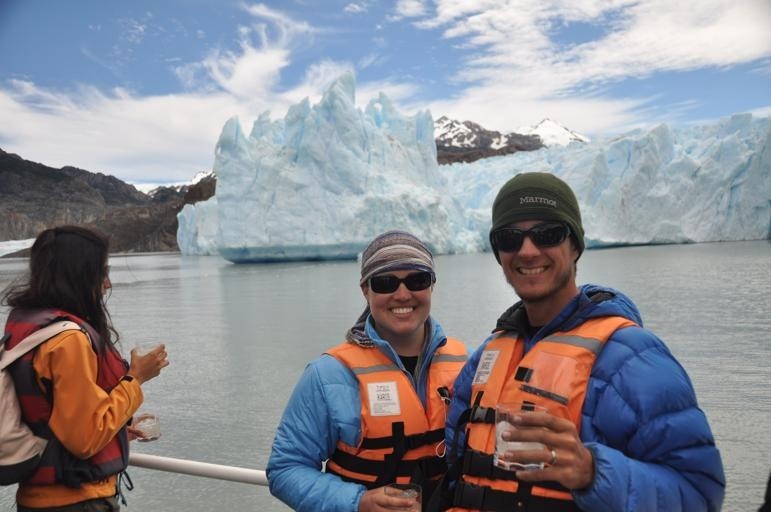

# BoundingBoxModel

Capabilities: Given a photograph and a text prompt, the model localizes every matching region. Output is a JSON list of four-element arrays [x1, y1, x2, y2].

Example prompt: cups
[[384, 483, 422, 512], [131, 412, 161, 442], [133, 340, 159, 355], [492, 403, 551, 473]]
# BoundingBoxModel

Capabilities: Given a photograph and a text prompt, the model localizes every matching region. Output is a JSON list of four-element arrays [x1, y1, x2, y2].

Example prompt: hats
[[360, 230, 435, 285], [489, 173, 584, 264]]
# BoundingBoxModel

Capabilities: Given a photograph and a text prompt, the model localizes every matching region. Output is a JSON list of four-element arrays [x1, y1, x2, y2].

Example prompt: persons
[[264, 229, 477, 512], [2, 226, 171, 511], [443, 171, 728, 512]]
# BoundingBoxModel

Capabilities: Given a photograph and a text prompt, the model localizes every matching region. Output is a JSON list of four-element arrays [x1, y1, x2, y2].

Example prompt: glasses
[[371, 272, 431, 292], [492, 221, 571, 252]]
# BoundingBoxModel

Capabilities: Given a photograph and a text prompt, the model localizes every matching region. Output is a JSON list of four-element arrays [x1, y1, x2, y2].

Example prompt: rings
[[549, 446, 559, 467]]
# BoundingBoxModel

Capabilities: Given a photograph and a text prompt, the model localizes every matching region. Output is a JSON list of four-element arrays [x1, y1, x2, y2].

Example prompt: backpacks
[[0, 320, 92, 486]]
